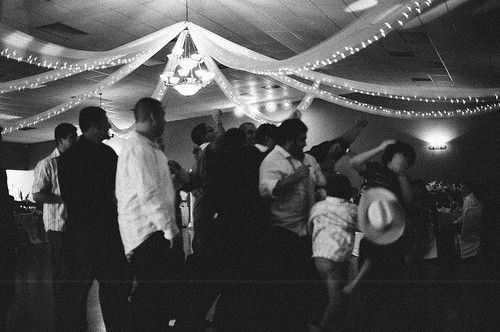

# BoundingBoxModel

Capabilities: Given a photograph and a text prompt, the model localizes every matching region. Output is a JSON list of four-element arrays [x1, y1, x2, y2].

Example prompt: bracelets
[[215, 120, 223, 126]]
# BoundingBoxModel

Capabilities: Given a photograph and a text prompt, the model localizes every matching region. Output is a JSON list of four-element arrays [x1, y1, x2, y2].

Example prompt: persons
[[0, 97, 500, 332]]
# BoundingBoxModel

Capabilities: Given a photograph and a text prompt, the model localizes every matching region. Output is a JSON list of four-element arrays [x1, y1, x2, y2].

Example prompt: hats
[[357, 187, 406, 246]]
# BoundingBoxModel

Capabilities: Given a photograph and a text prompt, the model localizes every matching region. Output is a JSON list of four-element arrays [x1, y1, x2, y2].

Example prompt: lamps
[[160, 0, 216, 97]]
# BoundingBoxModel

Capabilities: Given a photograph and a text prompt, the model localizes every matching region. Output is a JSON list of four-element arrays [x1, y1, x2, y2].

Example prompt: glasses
[[204, 127, 215, 134]]
